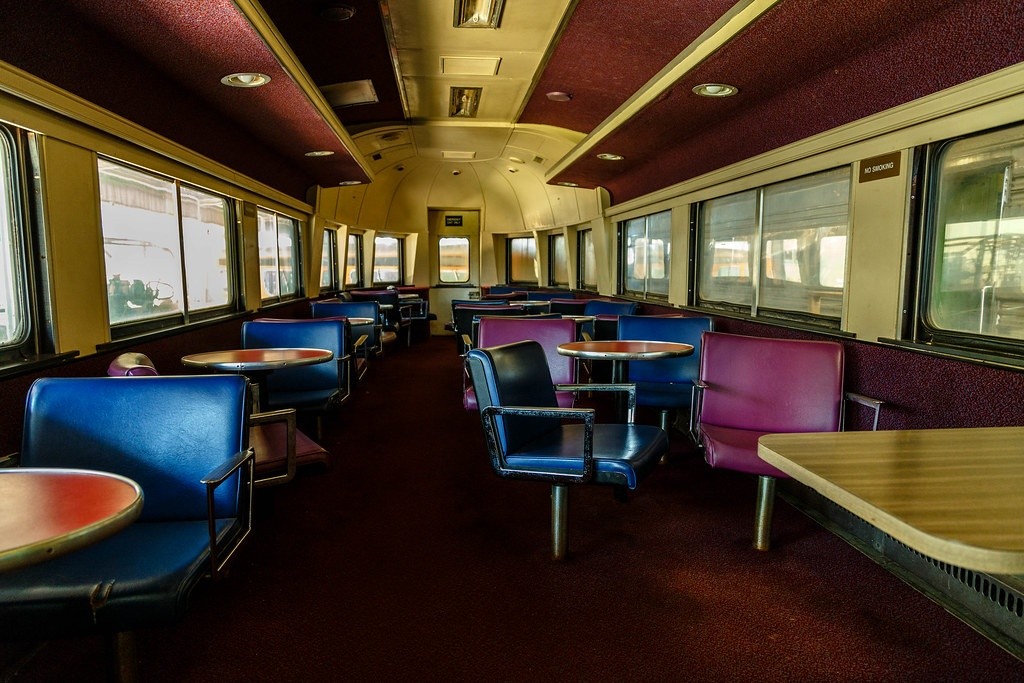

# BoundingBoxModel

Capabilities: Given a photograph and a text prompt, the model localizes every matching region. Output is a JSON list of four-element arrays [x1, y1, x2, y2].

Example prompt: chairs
[[0, 376, 255, 683], [462, 340, 670, 562], [107, 352, 331, 487], [241, 285, 437, 444], [690, 330, 885, 553], [443, 287, 714, 473]]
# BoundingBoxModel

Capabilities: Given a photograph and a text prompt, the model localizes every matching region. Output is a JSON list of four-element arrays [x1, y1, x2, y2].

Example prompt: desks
[[398, 293, 419, 298], [758, 426, 1024, 575], [0, 466, 145, 575], [182, 348, 334, 427], [560, 314, 595, 401], [379, 304, 393, 310], [509, 300, 550, 314], [557, 340, 696, 501], [347, 317, 375, 326]]
